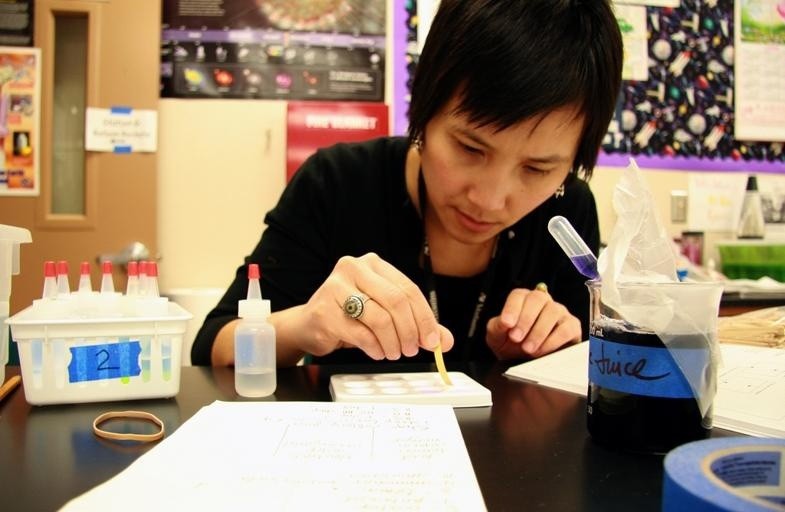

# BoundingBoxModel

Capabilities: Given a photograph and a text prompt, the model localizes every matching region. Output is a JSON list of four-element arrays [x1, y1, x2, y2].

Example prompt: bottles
[[38, 260, 163, 321], [232, 262, 278, 399], [737, 174, 766, 239], [547, 214, 604, 280], [681, 231, 703, 270]]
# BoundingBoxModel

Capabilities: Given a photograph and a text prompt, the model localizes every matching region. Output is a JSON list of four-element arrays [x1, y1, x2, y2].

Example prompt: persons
[[186, 1, 628, 373]]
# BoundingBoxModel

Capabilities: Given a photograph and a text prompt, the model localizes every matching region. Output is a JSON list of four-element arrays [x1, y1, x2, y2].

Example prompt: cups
[[670, 190, 687, 224], [583, 280, 719, 457]]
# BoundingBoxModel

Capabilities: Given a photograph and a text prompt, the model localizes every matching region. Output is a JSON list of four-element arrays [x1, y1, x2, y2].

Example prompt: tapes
[[661, 436, 785, 512]]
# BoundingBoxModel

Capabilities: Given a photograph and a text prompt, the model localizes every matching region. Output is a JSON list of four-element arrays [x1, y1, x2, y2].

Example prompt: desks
[[1, 307, 785, 511]]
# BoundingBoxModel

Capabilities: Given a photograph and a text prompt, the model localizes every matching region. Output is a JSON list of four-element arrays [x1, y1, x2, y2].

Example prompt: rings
[[342, 293, 373, 321]]
